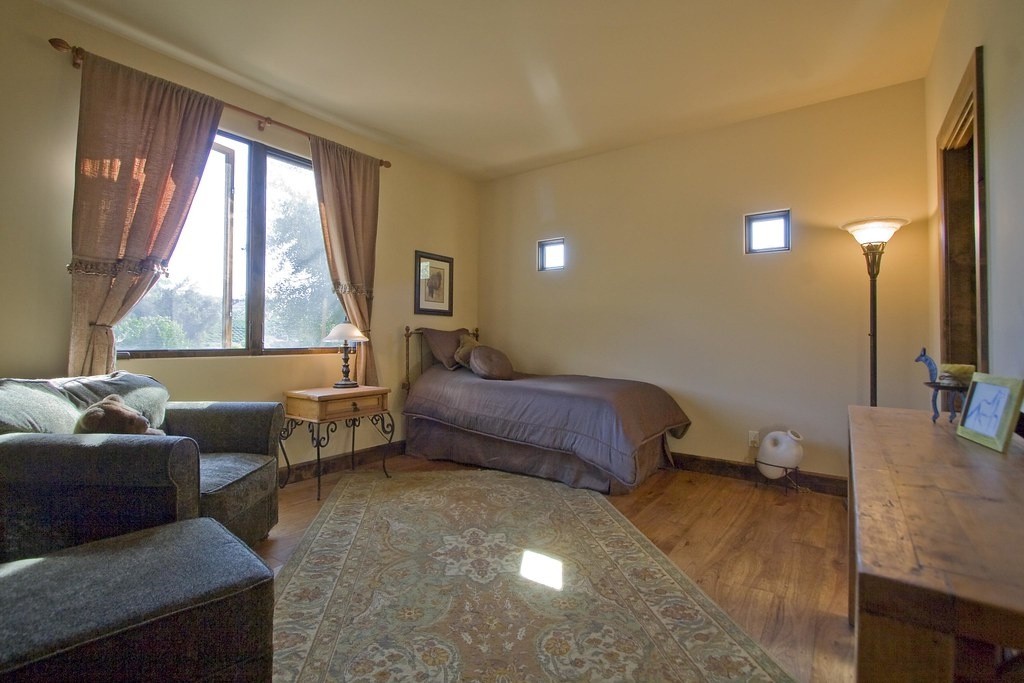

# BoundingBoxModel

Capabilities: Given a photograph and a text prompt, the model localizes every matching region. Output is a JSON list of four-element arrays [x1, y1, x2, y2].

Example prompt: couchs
[[0, 370, 284, 563]]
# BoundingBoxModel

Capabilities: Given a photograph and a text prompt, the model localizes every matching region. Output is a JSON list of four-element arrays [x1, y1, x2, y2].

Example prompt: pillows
[[470, 346, 513, 381], [454, 334, 481, 369], [415, 328, 470, 370]]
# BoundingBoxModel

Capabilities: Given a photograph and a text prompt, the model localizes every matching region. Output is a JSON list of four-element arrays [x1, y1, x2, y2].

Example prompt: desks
[[279, 385, 395, 501], [848, 405, 1024, 683]]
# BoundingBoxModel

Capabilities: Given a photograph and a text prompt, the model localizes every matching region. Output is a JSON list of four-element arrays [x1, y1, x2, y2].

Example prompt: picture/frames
[[414, 250, 454, 316], [957, 372, 1024, 454]]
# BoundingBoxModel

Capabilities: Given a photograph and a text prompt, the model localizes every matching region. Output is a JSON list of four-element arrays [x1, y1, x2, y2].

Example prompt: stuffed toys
[[73, 393, 166, 437]]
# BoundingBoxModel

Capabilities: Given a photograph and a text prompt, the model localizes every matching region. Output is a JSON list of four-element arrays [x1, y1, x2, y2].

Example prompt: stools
[[1, 516, 273, 683]]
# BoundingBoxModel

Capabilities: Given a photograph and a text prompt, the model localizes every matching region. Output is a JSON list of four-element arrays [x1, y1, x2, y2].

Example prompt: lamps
[[841, 217, 911, 408], [323, 316, 369, 389]]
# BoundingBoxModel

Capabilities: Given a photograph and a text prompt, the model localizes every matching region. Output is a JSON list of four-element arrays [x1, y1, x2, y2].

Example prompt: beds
[[402, 326, 691, 495]]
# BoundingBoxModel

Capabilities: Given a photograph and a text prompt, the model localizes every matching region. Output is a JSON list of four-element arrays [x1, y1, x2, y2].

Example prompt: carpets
[[273, 470, 801, 683]]
